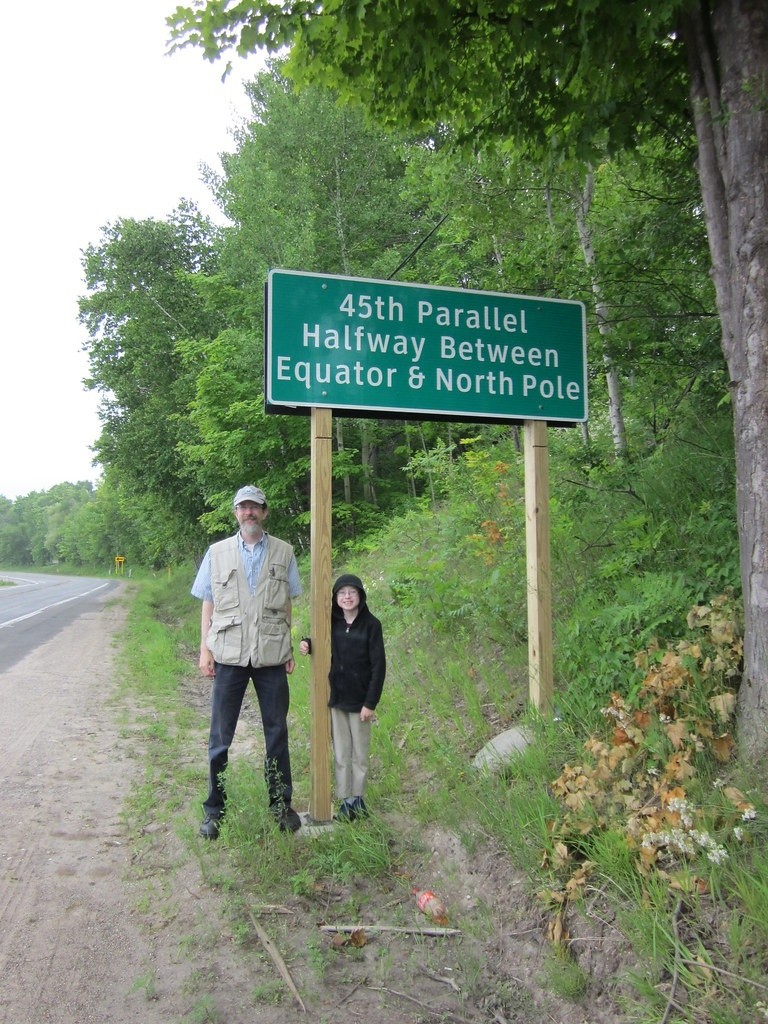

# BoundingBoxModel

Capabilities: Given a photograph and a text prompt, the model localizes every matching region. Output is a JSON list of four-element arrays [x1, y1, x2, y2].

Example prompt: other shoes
[[353, 798, 367, 819], [337, 803, 353, 821]]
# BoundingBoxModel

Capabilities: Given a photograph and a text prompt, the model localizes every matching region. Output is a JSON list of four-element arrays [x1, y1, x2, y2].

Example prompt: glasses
[[336, 589, 359, 597], [236, 504, 263, 511]]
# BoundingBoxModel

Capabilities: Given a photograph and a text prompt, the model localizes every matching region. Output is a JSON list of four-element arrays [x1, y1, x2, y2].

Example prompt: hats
[[234, 485, 268, 508]]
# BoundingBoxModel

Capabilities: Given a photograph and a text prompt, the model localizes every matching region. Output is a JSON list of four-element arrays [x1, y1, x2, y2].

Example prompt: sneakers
[[201, 811, 222, 836], [274, 807, 300, 832]]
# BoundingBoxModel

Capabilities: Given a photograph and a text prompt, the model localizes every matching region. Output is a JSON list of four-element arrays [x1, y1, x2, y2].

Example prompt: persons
[[190, 486, 302, 840], [300, 574, 386, 824]]
